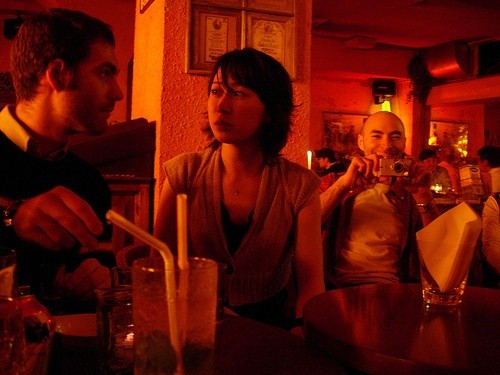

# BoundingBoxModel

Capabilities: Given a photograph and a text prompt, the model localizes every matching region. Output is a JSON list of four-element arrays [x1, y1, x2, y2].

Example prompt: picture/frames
[[430, 119, 471, 158], [182, 0, 302, 84], [321, 111, 370, 155], [140, 0, 155, 14]]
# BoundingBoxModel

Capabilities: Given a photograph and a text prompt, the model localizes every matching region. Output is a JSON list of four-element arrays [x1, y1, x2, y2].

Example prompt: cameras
[[379, 159, 410, 176]]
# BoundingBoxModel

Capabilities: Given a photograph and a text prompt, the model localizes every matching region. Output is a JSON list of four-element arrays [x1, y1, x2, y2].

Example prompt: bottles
[[0, 285, 56, 375]]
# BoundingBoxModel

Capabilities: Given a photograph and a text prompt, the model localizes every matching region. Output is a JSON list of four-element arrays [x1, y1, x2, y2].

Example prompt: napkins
[[413, 200, 482, 293]]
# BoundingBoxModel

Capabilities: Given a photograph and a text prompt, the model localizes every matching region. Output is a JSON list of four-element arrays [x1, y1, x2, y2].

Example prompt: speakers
[[372, 80, 395, 97]]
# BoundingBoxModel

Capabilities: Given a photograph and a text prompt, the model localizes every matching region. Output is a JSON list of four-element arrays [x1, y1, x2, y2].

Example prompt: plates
[[54, 313, 98, 344]]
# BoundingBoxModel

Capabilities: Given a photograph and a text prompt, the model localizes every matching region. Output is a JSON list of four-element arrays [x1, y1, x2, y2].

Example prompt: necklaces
[[224, 163, 246, 195]]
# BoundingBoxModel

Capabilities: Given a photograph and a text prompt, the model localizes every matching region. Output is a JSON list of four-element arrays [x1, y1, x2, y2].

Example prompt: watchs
[[415, 201, 436, 213]]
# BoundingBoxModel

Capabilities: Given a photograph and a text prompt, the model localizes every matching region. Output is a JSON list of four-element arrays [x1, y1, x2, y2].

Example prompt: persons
[[154, 48, 500, 340], [0, 9, 125, 304]]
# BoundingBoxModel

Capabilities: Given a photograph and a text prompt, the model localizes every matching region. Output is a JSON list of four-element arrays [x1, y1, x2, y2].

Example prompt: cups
[[93, 284, 136, 375], [0, 248, 16, 298], [415, 237, 475, 307], [130, 256, 219, 375]]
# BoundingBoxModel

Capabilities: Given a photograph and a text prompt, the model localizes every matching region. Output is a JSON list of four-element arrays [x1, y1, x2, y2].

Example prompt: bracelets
[[293, 316, 304, 327], [4, 199, 26, 241]]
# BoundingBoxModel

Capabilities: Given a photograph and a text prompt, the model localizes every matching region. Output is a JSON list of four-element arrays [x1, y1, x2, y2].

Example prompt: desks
[[101, 172, 153, 250], [302, 280, 500, 375], [45, 307, 345, 375]]
